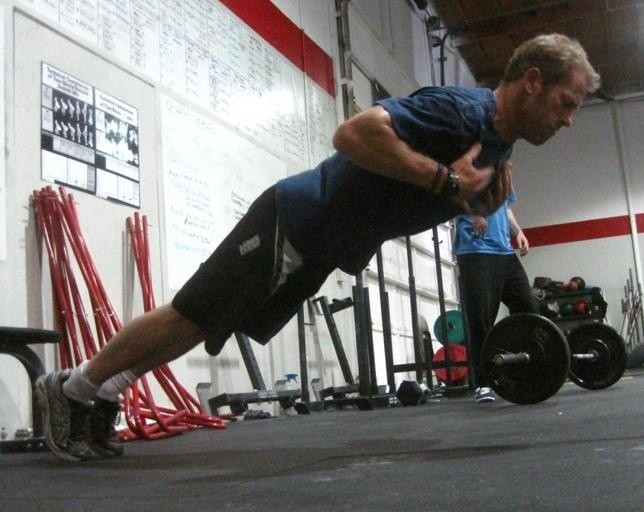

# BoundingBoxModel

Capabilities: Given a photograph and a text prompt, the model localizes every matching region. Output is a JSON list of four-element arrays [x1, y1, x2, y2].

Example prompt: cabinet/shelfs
[[540, 286, 602, 322]]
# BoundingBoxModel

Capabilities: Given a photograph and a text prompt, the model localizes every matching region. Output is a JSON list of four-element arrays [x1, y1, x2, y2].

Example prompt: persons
[[452, 183, 540, 403], [33, 32, 602, 463]]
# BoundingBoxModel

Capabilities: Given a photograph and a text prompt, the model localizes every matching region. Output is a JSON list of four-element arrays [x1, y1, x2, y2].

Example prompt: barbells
[[479, 313, 627, 405]]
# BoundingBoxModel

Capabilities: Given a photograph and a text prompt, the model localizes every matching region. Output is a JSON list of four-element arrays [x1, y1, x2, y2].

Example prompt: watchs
[[441, 163, 459, 201]]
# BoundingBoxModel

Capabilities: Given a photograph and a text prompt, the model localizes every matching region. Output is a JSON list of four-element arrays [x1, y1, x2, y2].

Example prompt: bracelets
[[429, 160, 442, 195]]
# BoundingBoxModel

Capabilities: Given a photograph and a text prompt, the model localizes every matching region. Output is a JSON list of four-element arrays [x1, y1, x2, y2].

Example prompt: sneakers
[[474, 386, 496, 404], [35, 368, 91, 463], [84, 395, 124, 459]]
